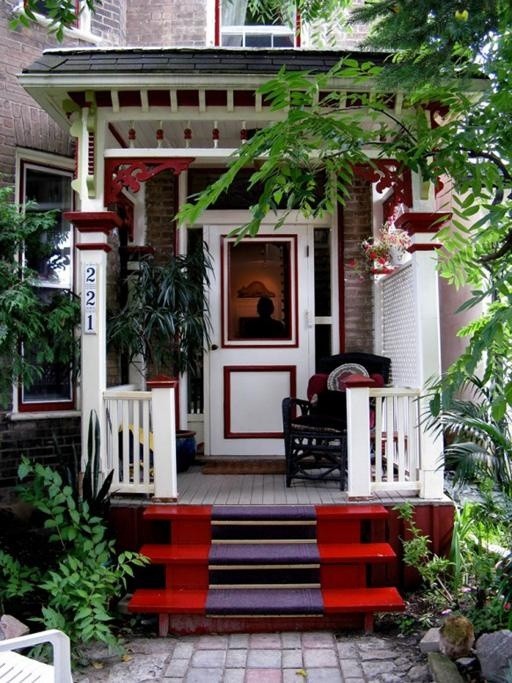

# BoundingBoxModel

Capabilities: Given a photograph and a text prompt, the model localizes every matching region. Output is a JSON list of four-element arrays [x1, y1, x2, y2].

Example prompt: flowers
[[348, 202, 411, 284]]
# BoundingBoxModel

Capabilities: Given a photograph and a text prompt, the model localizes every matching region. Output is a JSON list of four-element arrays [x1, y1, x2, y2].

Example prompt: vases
[[389, 246, 403, 265], [176, 430, 198, 473]]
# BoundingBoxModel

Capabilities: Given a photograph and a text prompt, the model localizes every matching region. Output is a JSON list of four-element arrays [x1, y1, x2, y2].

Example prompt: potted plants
[[105, 239, 218, 389]]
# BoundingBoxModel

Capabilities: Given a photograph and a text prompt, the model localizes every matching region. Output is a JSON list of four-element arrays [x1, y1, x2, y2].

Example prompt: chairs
[[283, 350, 391, 490], [0, 629, 74, 683]]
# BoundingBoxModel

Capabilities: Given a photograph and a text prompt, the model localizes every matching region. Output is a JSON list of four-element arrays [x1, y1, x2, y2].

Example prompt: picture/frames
[[219, 234, 300, 349]]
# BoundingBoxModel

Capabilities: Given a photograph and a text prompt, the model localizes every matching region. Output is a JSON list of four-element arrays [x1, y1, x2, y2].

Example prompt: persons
[[237, 297, 286, 339]]
